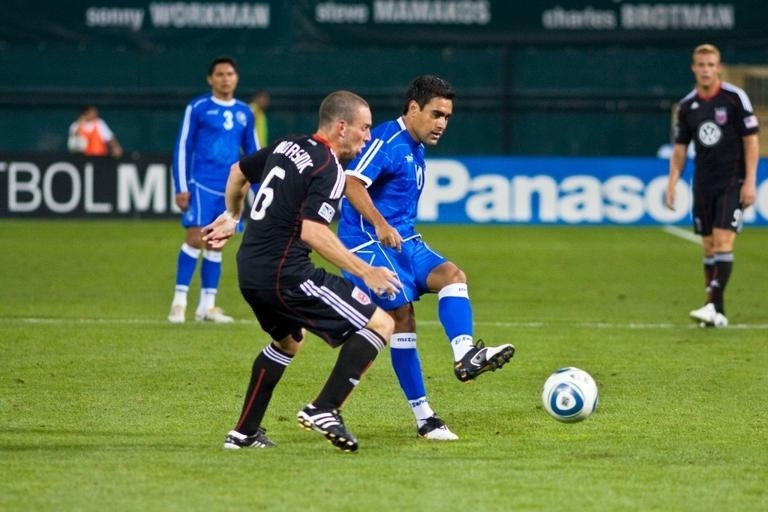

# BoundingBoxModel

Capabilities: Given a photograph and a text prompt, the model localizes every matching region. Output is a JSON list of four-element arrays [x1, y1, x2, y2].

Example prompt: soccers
[[542, 366, 598, 424]]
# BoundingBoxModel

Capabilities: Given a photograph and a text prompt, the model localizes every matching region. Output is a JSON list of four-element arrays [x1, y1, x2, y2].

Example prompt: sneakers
[[417, 418, 457, 443], [689, 304, 719, 323], [226, 429, 274, 451], [297, 401, 358, 455], [170, 290, 186, 327], [451, 343, 513, 382], [198, 307, 231, 324]]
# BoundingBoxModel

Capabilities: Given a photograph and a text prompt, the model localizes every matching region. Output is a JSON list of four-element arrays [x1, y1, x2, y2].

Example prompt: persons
[[666, 44, 759, 328], [338, 73, 516, 441], [67, 106, 122, 159], [201, 93, 403, 453], [232, 89, 270, 232], [168, 58, 262, 323]]
